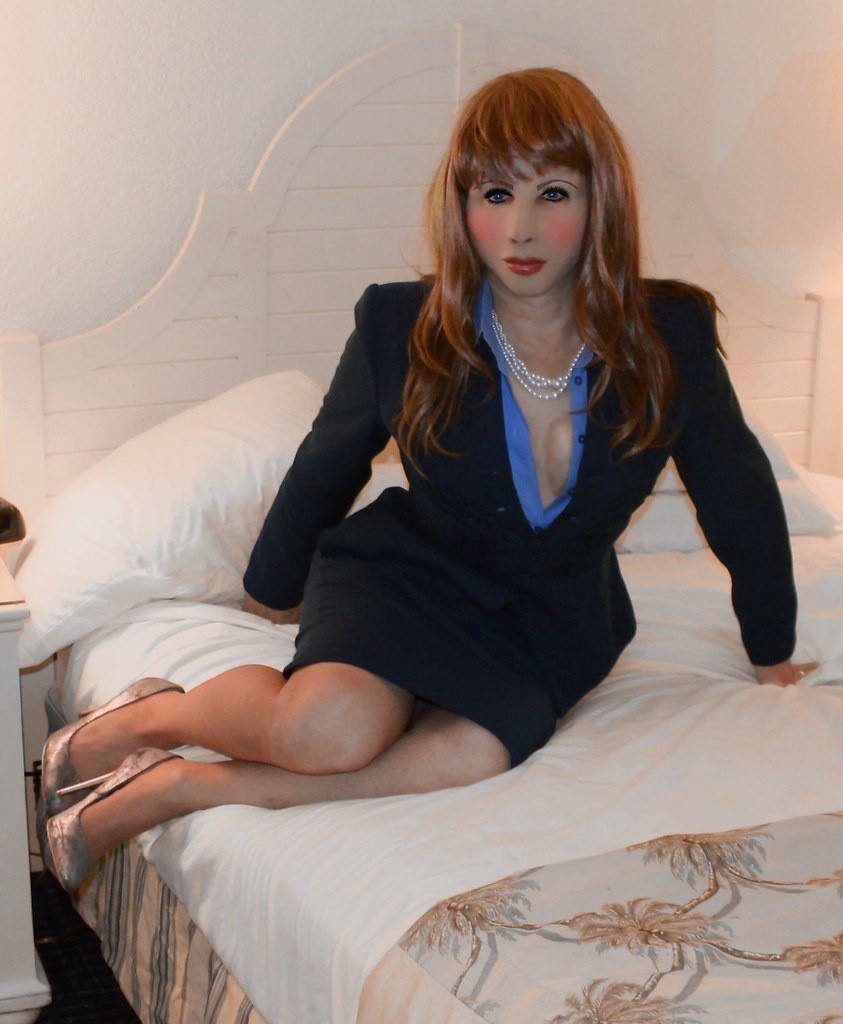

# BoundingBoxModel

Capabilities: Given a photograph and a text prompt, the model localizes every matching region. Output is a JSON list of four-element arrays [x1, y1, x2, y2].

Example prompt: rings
[[800, 670, 806, 677]]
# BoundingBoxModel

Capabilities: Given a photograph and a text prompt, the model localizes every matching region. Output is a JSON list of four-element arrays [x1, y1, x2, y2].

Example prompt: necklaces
[[491, 308, 585, 400]]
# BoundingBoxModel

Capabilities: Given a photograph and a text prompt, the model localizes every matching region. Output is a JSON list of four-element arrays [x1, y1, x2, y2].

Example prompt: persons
[[39, 68, 820, 893]]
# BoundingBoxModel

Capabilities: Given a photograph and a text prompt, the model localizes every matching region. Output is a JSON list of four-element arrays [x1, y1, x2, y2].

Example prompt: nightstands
[[0, 560, 54, 1024]]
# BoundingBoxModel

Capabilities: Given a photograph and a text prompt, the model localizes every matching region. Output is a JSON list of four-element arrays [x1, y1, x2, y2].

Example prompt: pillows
[[6, 368, 325, 667], [614, 417, 843, 555]]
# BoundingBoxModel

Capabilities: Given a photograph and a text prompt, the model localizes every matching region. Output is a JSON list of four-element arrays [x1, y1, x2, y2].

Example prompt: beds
[[0, 19, 843, 1024]]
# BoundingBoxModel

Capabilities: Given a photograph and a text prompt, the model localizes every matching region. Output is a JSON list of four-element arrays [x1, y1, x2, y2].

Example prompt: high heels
[[42, 675, 187, 814], [47, 746, 183, 890]]
